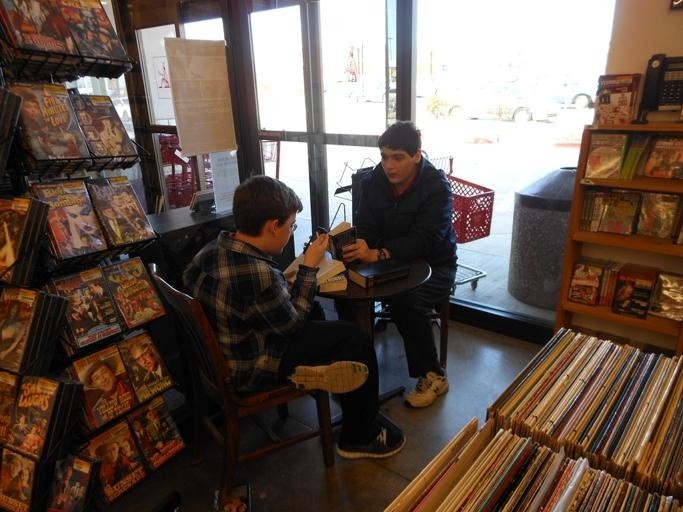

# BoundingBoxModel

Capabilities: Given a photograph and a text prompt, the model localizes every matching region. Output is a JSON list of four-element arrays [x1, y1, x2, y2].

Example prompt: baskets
[[444, 174, 493, 243]]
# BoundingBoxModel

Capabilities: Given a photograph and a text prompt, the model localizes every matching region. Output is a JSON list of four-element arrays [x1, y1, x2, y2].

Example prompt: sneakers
[[406, 368, 450, 409], [335, 423, 406, 459], [286, 360, 369, 394]]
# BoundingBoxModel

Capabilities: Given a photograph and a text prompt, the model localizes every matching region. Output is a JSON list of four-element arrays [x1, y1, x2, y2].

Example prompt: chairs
[[372, 297, 450, 369], [148, 263, 335, 512]]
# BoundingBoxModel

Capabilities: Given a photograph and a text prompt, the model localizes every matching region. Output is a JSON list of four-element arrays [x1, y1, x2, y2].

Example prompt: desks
[[316, 258, 432, 427]]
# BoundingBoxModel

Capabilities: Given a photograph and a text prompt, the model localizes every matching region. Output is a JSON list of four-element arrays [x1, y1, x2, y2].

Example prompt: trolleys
[[421, 151, 494, 293]]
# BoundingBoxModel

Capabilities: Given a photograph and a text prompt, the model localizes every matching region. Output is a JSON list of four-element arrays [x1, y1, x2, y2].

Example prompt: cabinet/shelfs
[[553, 123, 683, 357], [0, 27, 186, 511]]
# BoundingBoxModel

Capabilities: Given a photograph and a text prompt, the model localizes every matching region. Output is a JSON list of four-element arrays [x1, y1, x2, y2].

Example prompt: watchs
[[377, 248, 386, 262]]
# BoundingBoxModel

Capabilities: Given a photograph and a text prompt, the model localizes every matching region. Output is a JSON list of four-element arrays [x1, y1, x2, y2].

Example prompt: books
[[379, 327, 683, 512], [2, 1, 183, 512], [327, 221, 357, 261], [281, 250, 345, 289], [317, 273, 347, 292], [213, 481, 253, 512], [347, 261, 409, 288], [566, 131, 683, 323], [592, 71, 640, 126]]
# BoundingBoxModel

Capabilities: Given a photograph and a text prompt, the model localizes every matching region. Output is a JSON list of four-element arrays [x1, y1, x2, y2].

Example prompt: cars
[[549, 83, 597, 109], [438, 83, 557, 122]]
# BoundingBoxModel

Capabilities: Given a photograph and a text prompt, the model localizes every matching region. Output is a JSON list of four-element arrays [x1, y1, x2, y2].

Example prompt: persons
[[185, 174, 405, 459], [341, 121, 458, 409]]
